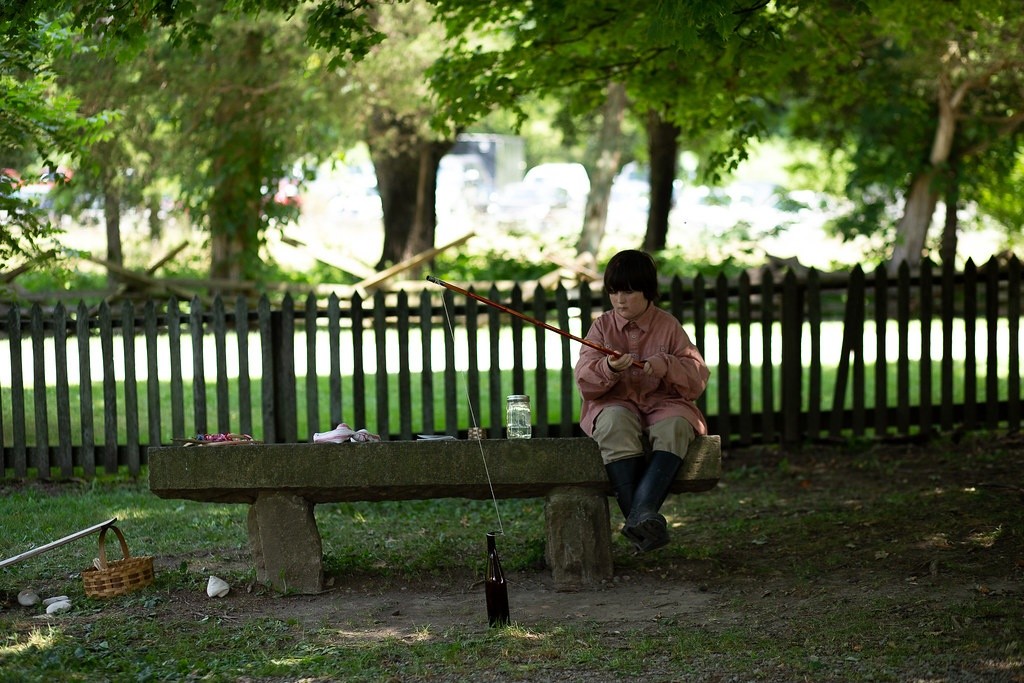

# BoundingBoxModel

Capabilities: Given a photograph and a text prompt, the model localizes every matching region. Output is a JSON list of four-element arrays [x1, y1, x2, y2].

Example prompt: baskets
[[82, 525, 156, 600]]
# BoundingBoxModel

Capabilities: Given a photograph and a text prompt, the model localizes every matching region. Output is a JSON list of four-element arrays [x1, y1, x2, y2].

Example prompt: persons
[[575, 252, 710, 552]]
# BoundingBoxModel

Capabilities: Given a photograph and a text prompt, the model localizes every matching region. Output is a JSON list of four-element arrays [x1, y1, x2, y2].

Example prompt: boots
[[620, 450, 684, 542], [604, 454, 671, 551]]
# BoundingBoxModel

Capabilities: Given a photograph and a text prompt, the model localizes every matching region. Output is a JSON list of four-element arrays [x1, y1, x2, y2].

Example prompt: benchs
[[148, 433, 721, 597]]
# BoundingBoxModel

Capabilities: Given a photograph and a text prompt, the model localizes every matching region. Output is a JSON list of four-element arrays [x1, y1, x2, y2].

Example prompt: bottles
[[506, 395, 532, 438], [485, 532, 511, 628]]
